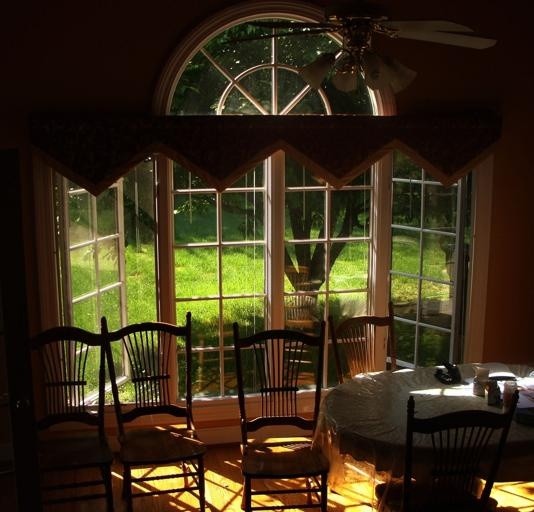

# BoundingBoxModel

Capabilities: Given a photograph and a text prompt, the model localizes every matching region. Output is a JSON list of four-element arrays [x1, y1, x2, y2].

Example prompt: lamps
[[299, 36, 418, 95]]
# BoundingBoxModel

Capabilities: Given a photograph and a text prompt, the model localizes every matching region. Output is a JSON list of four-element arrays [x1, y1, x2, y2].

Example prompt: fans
[[221, 0, 498, 50]]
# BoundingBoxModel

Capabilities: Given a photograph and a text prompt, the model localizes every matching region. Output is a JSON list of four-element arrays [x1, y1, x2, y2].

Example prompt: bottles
[[503, 381, 517, 408]]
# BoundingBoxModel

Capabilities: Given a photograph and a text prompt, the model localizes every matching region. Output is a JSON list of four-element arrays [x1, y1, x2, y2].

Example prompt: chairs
[[101, 312, 207, 512], [328, 300, 398, 384], [375, 390, 520, 512], [24, 313, 115, 512], [233, 320, 330, 512]]
